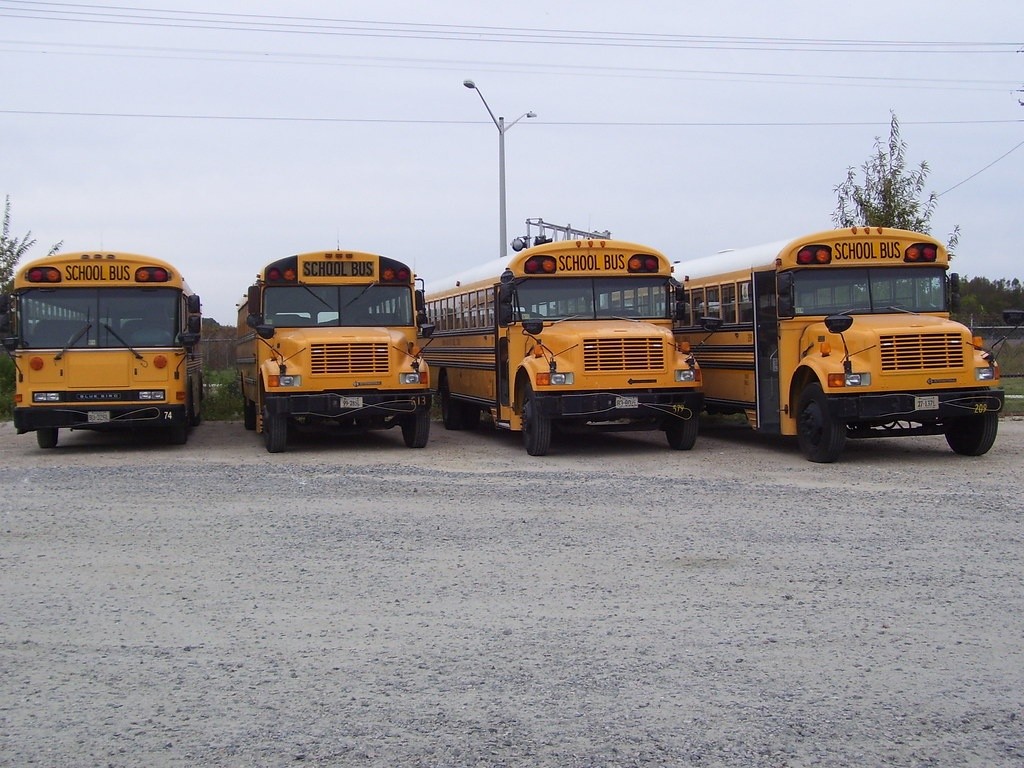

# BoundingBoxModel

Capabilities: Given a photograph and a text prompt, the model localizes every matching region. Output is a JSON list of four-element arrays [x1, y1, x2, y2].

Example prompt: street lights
[[462, 79, 535, 257]]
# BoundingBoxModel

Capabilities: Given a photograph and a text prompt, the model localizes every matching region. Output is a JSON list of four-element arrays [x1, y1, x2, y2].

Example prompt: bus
[[3, 252, 205, 449], [607, 225, 1023, 464], [236, 250, 434, 454], [371, 236, 724, 459]]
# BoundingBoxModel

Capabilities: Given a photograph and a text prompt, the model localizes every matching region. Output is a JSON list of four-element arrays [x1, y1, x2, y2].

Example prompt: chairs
[[742, 306, 776, 345], [123, 316, 174, 334], [32, 317, 105, 340]]
